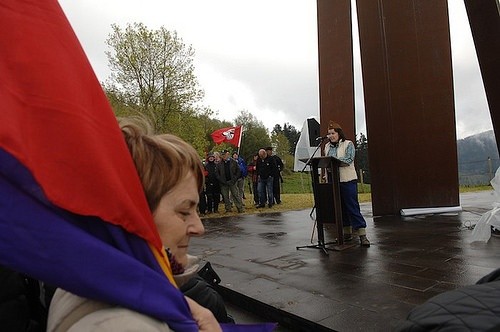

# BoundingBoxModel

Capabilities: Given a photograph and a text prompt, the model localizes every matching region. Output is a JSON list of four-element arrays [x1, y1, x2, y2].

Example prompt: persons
[[247, 147, 284, 208], [321, 121, 371, 246], [0, 123, 247, 332]]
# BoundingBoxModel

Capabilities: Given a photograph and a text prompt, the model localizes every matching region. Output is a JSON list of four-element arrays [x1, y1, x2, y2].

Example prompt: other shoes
[[361, 238, 370, 245], [238, 210, 245, 213], [208, 211, 212, 214], [226, 211, 232, 212], [344, 235, 352, 240], [214, 211, 220, 214], [269, 204, 272, 208], [256, 205, 265, 208]]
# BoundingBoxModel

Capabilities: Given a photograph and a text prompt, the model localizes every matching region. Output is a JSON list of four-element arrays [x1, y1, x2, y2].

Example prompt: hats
[[220, 150, 228, 155], [206, 154, 215, 158], [328, 120, 341, 129]]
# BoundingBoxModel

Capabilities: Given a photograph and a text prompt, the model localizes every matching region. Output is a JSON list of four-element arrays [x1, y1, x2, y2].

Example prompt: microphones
[[315, 136, 331, 140]]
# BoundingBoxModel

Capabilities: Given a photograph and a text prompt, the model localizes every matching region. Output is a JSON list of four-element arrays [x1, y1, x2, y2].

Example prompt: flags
[[210, 123, 243, 148], [0, 0, 278, 332]]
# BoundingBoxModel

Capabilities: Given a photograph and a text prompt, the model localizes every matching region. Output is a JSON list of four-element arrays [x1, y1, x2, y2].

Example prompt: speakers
[[195, 259, 221, 289], [307, 118, 321, 147]]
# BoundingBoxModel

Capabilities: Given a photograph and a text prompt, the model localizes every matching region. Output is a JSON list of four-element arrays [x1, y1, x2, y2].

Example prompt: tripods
[[296, 142, 338, 257]]
